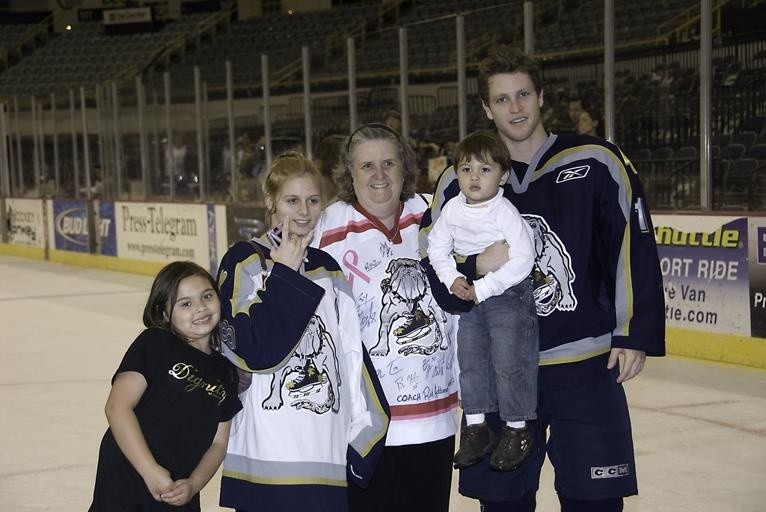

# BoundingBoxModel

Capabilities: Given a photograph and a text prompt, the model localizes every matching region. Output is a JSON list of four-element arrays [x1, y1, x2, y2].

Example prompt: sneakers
[[487, 423, 538, 472], [452, 421, 500, 472]]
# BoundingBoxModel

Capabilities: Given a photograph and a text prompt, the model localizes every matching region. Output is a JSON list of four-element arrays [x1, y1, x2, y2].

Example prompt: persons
[[382, 98, 603, 192], [90, 262, 244, 510], [426, 130, 538, 471], [148, 124, 273, 208], [314, 125, 459, 512], [212, 156, 365, 510], [417, 45, 665, 511], [34, 151, 116, 202]]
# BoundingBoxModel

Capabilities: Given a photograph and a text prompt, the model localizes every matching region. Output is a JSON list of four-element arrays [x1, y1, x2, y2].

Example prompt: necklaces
[[358, 201, 408, 243]]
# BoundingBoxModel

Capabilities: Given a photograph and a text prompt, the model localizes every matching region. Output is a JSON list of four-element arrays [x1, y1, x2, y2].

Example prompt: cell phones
[[267, 223, 309, 263]]
